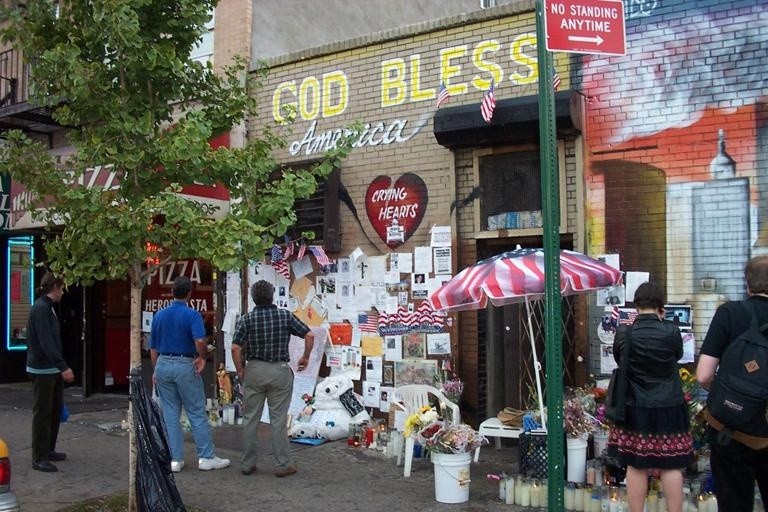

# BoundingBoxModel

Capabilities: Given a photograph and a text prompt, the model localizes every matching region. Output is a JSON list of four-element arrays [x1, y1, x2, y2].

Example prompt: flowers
[[592, 386, 608, 401], [680, 368, 702, 433], [402, 405, 435, 437], [423, 423, 443, 450], [596, 406, 608, 428], [442, 379, 462, 401], [563, 401, 590, 437]]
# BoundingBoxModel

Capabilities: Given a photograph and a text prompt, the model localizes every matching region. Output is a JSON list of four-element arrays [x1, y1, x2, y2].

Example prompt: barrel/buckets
[[431, 450, 471, 504], [566, 437, 588, 482], [592, 430, 611, 457]]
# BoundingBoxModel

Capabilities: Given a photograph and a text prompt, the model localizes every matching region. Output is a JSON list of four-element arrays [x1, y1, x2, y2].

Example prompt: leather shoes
[[274, 464, 296, 477], [47, 451, 67, 460], [241, 464, 256, 474], [31, 459, 58, 472]]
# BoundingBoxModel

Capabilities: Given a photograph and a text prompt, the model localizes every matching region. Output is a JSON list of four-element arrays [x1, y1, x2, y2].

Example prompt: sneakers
[[171, 460, 185, 472], [197, 455, 231, 470]]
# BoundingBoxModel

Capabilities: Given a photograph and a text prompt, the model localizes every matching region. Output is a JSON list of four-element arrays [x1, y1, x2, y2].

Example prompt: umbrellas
[[428, 243, 623, 429]]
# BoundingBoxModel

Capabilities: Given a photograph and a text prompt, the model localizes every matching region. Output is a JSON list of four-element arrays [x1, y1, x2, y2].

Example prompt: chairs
[[394, 384, 460, 477]]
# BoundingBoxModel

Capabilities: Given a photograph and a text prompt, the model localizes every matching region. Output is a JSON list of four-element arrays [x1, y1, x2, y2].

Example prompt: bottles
[[692, 438, 712, 473], [641, 472, 720, 512], [346, 419, 389, 450], [499, 472, 548, 509], [205, 398, 243, 429], [564, 457, 628, 512]]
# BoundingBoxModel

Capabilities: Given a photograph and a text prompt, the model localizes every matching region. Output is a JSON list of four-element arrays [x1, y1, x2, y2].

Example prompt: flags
[[359, 314, 378, 333], [271, 239, 330, 280], [436, 67, 562, 123], [619, 312, 638, 324], [327, 356, 342, 366]]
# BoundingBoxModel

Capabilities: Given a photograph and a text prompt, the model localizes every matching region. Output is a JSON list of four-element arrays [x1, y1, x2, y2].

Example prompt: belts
[[160, 351, 197, 359]]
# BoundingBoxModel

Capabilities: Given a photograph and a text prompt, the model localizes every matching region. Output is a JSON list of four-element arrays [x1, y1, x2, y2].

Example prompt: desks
[[474, 416, 536, 465]]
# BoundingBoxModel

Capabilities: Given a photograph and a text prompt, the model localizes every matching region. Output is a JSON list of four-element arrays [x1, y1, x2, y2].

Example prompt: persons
[[233, 280, 315, 476], [149, 277, 230, 470], [27, 277, 75, 470], [613, 284, 694, 512], [695, 256, 767, 511]]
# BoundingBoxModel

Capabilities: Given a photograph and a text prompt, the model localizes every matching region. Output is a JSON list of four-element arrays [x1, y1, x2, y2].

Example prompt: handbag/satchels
[[59, 405, 69, 423], [602, 368, 629, 426]]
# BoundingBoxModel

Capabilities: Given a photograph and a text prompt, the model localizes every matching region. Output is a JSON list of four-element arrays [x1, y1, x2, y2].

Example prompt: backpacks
[[702, 330, 768, 452]]
